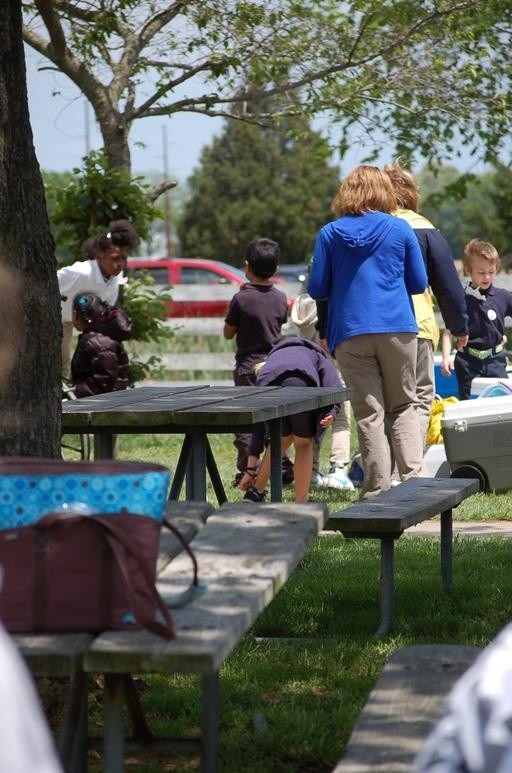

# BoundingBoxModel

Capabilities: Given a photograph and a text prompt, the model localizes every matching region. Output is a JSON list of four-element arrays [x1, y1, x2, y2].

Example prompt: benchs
[[8, 501, 217, 773], [61, 379, 90, 463], [82, 501, 331, 773], [327, 477, 481, 643], [333, 644, 485, 773]]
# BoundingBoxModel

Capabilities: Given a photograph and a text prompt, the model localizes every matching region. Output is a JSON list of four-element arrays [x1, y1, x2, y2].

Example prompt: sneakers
[[243, 486, 268, 502], [316, 464, 362, 494]]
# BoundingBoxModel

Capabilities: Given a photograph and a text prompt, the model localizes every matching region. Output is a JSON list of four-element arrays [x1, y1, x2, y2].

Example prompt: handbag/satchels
[[0, 458, 169, 629]]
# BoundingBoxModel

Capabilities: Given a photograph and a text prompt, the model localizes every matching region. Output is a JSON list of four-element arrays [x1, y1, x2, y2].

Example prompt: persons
[[237, 335, 344, 505], [56, 218, 140, 381], [306, 164, 430, 501], [290, 293, 355, 492], [222, 237, 294, 487], [441, 236, 512, 402], [68, 291, 134, 462], [348, 155, 468, 486]]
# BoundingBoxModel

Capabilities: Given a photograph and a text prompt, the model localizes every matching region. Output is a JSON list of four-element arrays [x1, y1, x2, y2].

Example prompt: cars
[[275, 262, 308, 281], [127, 256, 303, 316]]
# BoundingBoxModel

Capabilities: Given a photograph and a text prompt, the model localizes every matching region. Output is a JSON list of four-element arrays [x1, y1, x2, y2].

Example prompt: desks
[[61, 384, 353, 505]]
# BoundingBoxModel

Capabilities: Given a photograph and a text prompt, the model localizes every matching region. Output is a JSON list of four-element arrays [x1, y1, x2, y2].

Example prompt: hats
[[291, 294, 318, 340]]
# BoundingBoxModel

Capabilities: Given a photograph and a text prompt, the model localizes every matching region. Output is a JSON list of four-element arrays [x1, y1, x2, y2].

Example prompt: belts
[[469, 345, 503, 359]]
